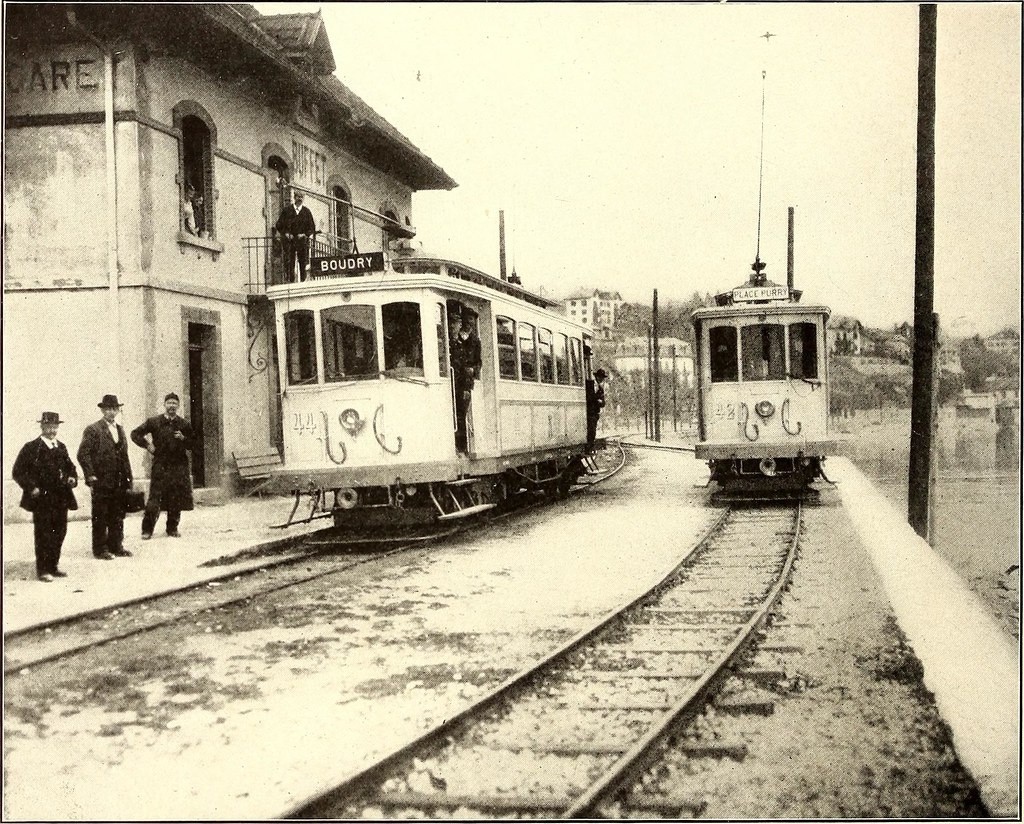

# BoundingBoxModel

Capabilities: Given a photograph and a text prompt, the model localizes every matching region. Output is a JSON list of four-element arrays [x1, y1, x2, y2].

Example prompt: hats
[[461, 307, 478, 320], [593, 369, 608, 378], [98, 394, 125, 408], [165, 392, 180, 401], [35, 411, 64, 424]]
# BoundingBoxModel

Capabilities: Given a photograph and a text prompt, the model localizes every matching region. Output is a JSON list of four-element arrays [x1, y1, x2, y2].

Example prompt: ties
[[51, 442, 57, 450]]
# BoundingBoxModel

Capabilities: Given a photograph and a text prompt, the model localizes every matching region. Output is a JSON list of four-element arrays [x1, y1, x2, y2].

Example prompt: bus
[[266, 250, 612, 548], [685, 272, 838, 504]]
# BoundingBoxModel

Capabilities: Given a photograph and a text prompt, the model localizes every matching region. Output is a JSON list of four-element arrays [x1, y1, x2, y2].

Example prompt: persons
[[76, 395, 134, 561], [712, 335, 737, 384], [131, 393, 196, 537], [448, 309, 482, 453], [183, 181, 205, 237], [12, 412, 79, 581], [586, 369, 609, 452], [270, 188, 315, 283]]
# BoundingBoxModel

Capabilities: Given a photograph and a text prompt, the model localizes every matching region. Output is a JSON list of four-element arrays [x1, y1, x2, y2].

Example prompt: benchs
[[231, 448, 288, 498]]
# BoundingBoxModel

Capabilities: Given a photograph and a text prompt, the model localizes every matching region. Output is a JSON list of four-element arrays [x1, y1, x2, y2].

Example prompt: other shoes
[[38, 573, 55, 582], [109, 548, 133, 557], [168, 530, 181, 537], [50, 569, 68, 578], [95, 552, 115, 560], [141, 531, 151, 540]]
